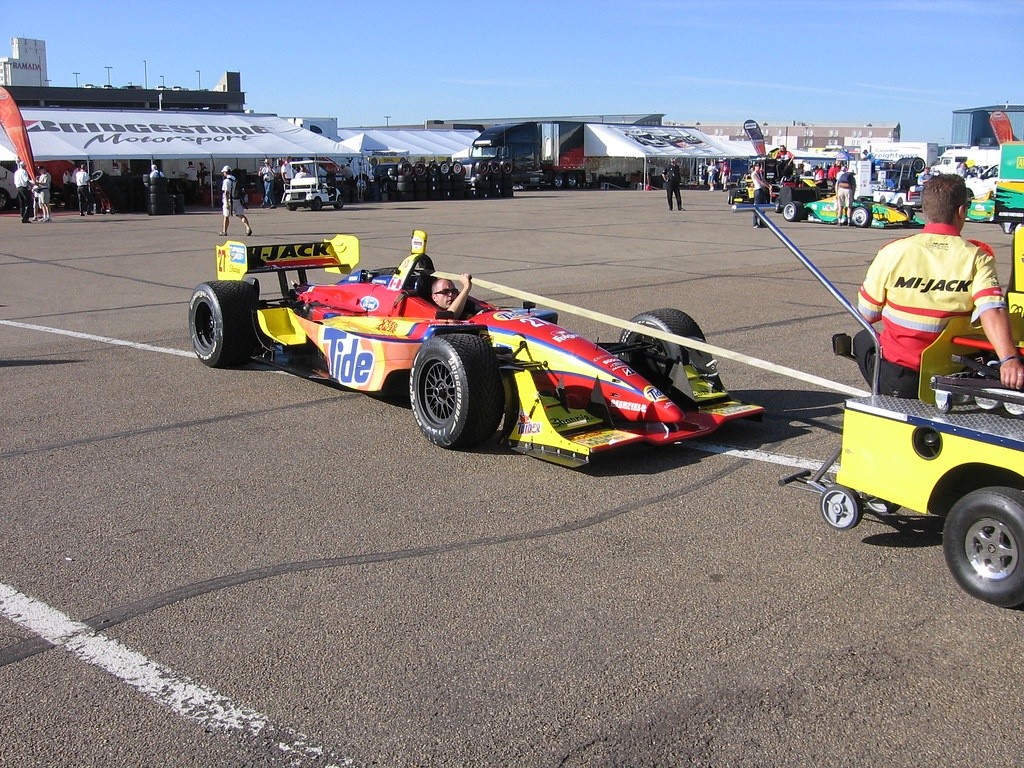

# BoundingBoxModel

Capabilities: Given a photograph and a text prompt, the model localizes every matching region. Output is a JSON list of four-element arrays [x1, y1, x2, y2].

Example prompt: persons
[[863, 150, 875, 162], [432, 273, 485, 320], [715, 163, 722, 189], [918, 167, 934, 186], [751, 162, 772, 228], [13, 158, 357, 237], [662, 157, 686, 211], [957, 163, 989, 179], [836, 169, 856, 226], [812, 162, 848, 193], [707, 161, 718, 192], [853, 174, 1024, 401], [775, 145, 794, 181], [719, 160, 732, 192]]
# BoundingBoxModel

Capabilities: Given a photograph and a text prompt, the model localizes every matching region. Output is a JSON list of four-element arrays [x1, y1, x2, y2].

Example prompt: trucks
[[731, 203, 1024, 609], [726, 141, 1023, 223]]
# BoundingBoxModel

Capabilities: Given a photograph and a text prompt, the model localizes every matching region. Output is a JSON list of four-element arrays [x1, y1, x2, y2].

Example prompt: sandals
[[244, 229, 252, 236], [218, 232, 227, 236]]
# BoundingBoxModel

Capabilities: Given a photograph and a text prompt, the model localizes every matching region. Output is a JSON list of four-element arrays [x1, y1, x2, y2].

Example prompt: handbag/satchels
[[241, 194, 248, 205]]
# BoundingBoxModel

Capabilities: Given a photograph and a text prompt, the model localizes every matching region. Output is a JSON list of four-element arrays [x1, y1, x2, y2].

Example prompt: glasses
[[964, 201, 972, 209], [434, 288, 458, 295]]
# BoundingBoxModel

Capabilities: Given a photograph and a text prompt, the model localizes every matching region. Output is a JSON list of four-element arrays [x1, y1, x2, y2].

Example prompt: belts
[[41, 188, 48, 189]]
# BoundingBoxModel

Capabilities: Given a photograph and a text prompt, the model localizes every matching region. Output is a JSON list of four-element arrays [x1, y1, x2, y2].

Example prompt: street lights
[[195, 70, 202, 92], [72, 73, 81, 88], [143, 60, 147, 89], [160, 75, 166, 90], [105, 66, 113, 89], [384, 115, 391, 127]]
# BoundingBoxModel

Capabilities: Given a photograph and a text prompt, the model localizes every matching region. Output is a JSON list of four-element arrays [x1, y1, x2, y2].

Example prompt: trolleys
[[86, 175, 116, 215]]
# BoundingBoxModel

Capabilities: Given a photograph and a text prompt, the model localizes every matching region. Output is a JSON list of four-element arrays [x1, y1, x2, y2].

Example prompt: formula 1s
[[187, 228, 767, 470], [780, 194, 925, 230]]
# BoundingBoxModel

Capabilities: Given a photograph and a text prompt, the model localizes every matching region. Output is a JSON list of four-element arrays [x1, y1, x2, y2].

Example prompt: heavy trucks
[[468, 121, 585, 190]]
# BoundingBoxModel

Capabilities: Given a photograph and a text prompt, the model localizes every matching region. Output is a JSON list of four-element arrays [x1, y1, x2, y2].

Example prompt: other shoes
[[271, 204, 276, 209], [33, 217, 37, 221], [80, 213, 85, 216], [761, 223, 768, 228], [43, 218, 51, 223], [87, 211, 94, 215], [754, 225, 761, 228], [22, 218, 31, 223]]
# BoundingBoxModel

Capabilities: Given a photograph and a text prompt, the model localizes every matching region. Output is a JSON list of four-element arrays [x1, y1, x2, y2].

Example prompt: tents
[[834, 149, 854, 167], [583, 123, 824, 191], [0, 108, 483, 206]]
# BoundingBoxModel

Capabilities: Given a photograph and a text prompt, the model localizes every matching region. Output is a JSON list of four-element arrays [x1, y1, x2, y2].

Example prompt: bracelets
[[1000, 355, 1019, 364]]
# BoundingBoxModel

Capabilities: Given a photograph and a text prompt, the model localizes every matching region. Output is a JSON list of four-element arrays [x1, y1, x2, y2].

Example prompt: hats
[[847, 169, 855, 174], [36, 165, 44, 170], [81, 164, 87, 168], [20, 161, 26, 167], [221, 166, 231, 172], [264, 159, 269, 163]]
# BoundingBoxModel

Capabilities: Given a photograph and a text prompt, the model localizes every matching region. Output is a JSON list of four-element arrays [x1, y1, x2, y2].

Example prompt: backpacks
[[228, 178, 245, 199]]
